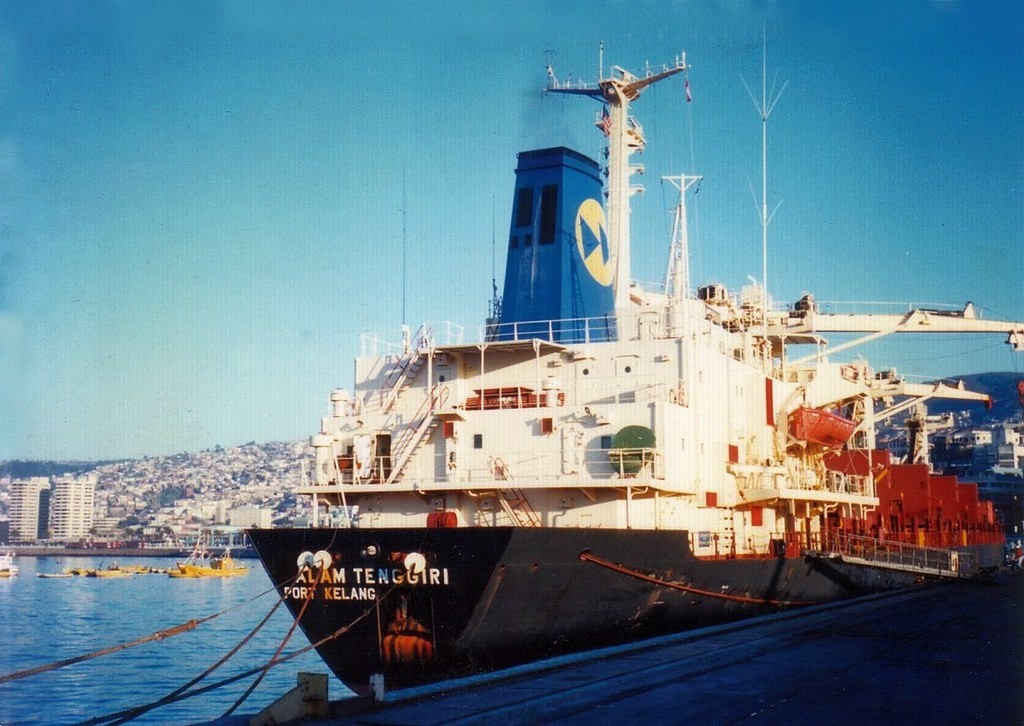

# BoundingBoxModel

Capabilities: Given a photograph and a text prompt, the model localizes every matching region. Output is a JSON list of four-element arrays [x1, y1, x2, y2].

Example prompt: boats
[[1, 564, 202, 581], [178, 528, 248, 576]]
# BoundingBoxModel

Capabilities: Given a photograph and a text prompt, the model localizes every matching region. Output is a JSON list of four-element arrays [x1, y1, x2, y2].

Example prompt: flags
[[548, 65, 553, 77], [686, 80, 691, 103]]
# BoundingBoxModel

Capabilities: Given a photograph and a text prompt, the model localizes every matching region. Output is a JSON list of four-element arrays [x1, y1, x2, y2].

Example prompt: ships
[[240, 46, 1021, 705]]
